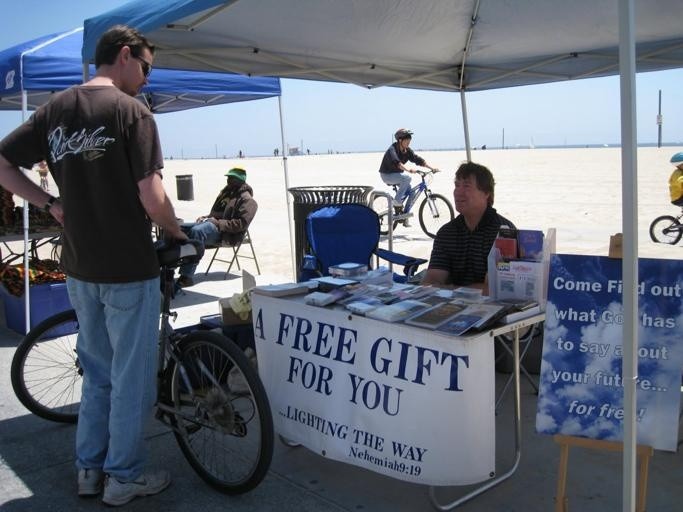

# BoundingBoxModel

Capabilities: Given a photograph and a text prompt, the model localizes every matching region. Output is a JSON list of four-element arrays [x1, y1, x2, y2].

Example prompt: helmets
[[671, 153, 683, 168], [394, 128, 415, 139]]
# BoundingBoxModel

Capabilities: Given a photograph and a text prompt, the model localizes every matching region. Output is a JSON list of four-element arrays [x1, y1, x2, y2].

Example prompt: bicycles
[[10, 232, 274, 495], [368, 168, 454, 238], [650, 203, 683, 245]]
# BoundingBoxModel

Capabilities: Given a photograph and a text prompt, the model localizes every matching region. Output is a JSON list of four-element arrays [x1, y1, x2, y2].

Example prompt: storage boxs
[[0, 282, 81, 341]]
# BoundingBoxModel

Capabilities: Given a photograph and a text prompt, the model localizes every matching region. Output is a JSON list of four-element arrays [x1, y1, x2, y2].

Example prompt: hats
[[224, 168, 247, 182]]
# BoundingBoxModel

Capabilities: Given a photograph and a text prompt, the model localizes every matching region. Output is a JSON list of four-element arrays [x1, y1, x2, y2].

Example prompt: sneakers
[[174, 275, 194, 289], [391, 199, 403, 208], [77, 465, 107, 498], [101, 461, 175, 506]]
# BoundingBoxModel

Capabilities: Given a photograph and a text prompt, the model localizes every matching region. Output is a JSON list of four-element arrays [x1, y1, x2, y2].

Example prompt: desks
[[249, 276, 548, 512], [0, 226, 63, 264]]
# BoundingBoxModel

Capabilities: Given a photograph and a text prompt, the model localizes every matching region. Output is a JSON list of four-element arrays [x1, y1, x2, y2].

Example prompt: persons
[[668, 152, 683, 206], [160, 168, 258, 289], [419, 163, 516, 291], [379, 128, 439, 227], [0, 26, 187, 507]]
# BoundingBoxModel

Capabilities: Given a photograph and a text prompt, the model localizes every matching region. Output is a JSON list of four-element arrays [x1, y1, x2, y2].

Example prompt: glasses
[[129, 49, 153, 79]]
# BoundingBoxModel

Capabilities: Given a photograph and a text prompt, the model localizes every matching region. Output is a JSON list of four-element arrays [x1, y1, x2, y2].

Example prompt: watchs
[[45, 196, 57, 213]]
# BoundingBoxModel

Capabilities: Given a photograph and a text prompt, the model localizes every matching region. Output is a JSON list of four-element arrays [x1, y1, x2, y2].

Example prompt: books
[[500, 298, 537, 311], [329, 262, 369, 276], [253, 283, 309, 298], [496, 271, 516, 302], [510, 261, 542, 304], [462, 303, 504, 328], [309, 275, 361, 293], [496, 304, 539, 324], [477, 300, 515, 333], [496, 236, 517, 262], [346, 300, 385, 315], [374, 277, 454, 306], [518, 228, 544, 262], [366, 300, 432, 323], [437, 312, 484, 337], [404, 301, 473, 329], [495, 259, 515, 271], [497, 227, 517, 238]]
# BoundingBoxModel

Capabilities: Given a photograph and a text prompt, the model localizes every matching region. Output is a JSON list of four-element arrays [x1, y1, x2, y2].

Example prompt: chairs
[[551, 431, 656, 512], [298, 202, 429, 284], [205, 228, 261, 281], [495, 322, 540, 403]]
